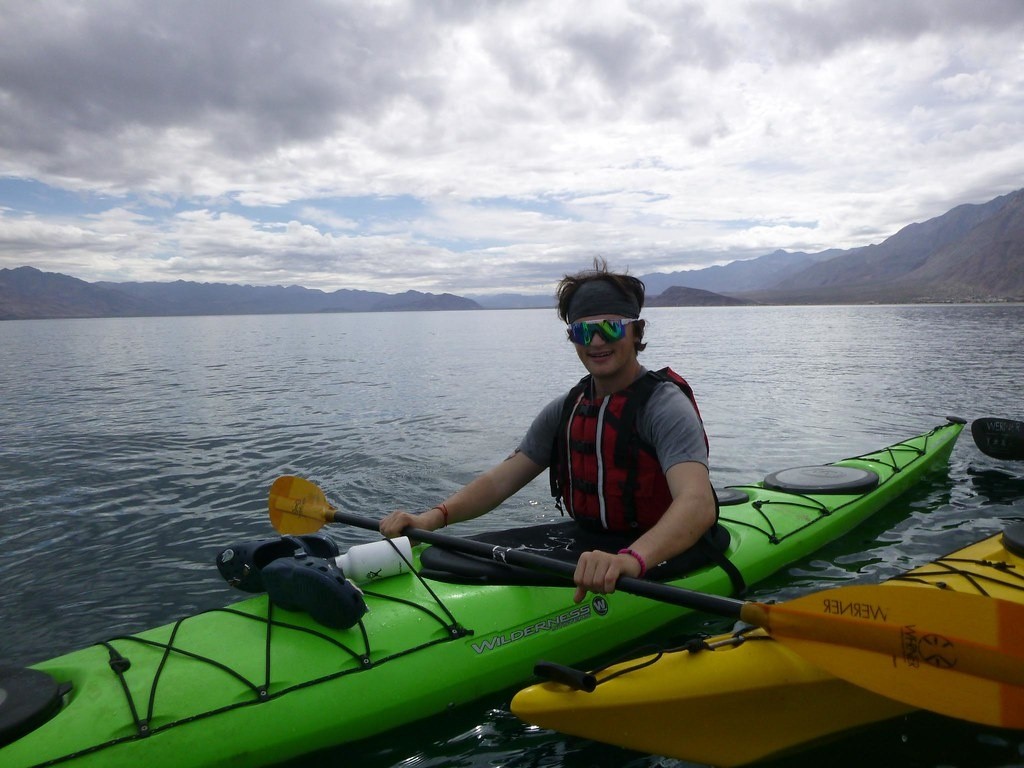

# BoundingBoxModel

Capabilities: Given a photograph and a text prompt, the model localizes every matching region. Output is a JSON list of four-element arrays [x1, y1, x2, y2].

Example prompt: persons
[[378, 263, 731, 603]]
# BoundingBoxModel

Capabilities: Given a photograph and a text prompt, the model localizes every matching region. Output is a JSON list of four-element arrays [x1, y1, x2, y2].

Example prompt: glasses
[[568, 318, 640, 347]]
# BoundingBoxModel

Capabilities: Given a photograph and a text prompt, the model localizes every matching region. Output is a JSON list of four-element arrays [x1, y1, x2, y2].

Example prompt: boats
[[0, 409, 966, 768], [506, 519, 1024, 768]]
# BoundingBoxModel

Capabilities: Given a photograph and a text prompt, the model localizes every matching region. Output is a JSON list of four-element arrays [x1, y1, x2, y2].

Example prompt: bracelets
[[618, 549, 646, 580], [432, 504, 449, 528]]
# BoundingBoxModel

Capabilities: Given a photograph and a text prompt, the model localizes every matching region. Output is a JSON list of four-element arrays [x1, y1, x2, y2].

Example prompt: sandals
[[216, 533, 339, 591], [261, 557, 369, 629]]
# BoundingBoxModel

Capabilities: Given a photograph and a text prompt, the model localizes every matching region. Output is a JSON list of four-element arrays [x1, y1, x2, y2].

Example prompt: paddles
[[267, 477, 1024, 731], [970, 417, 1024, 462]]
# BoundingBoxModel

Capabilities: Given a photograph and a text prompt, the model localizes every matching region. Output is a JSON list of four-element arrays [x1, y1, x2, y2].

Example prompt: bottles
[[333, 535, 414, 584]]
